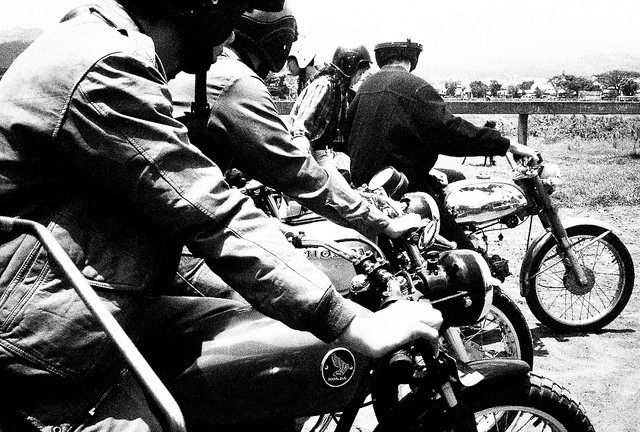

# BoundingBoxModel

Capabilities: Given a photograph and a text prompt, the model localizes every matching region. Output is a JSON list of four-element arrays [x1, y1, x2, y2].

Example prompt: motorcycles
[[276, 165, 532, 431], [154, 234, 595, 431], [443, 152, 634, 334]]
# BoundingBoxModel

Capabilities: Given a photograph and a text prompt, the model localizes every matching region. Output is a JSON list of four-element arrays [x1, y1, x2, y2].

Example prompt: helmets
[[168, 0, 285, 48], [330, 44, 373, 78], [232, 5, 299, 44], [287, 42, 317, 69], [374, 38, 423, 72]]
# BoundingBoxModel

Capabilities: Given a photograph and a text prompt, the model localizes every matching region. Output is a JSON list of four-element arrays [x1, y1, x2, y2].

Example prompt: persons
[[1, 0, 443, 432], [284, 42, 373, 160], [165, 0, 423, 299], [286, 38, 321, 98], [341, 41, 539, 256]]
